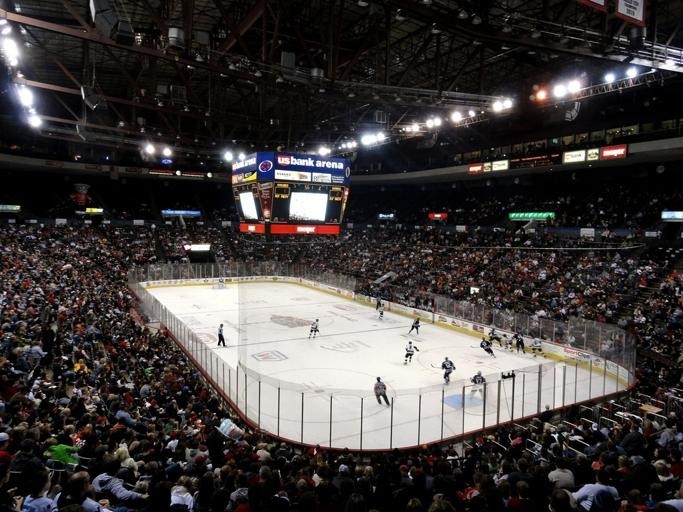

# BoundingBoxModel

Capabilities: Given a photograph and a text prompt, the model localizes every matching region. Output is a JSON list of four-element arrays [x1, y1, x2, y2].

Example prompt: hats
[[338, 464, 351, 475], [115, 410, 137, 425], [355, 464, 375, 476], [256, 442, 268, 450], [134, 438, 155, 455], [316, 464, 331, 479], [517, 451, 534, 471], [0, 432, 14, 442], [189, 428, 209, 468], [95, 443, 109, 454]]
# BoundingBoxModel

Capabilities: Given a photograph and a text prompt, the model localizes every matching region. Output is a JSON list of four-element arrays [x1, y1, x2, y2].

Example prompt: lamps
[[74, 39, 100, 142]]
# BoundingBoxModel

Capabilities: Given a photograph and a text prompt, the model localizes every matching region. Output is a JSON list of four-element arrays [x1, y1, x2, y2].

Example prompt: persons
[[0, 188, 683, 512]]
